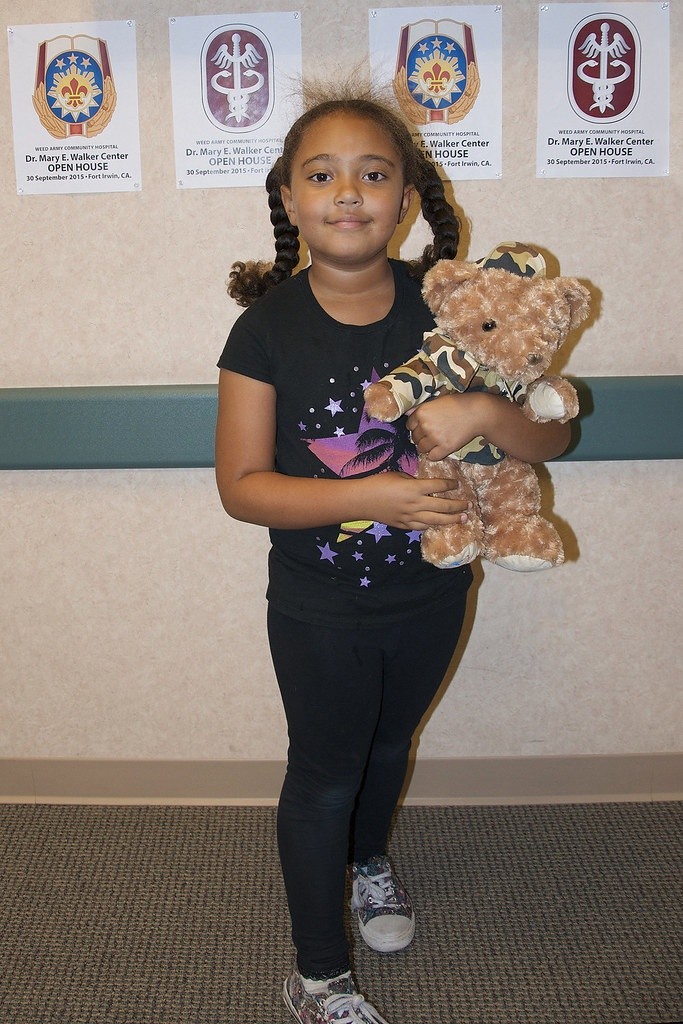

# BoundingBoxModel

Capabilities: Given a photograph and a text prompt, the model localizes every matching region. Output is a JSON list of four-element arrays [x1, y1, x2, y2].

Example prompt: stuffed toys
[[362, 240, 593, 572]]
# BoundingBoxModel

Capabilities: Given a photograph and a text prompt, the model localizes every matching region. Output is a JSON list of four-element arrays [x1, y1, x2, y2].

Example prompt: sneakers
[[346, 855, 417, 953], [282, 959, 388, 1024]]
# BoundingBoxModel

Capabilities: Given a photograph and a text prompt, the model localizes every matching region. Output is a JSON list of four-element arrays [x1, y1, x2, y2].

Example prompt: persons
[[214, 48, 582, 1024]]
